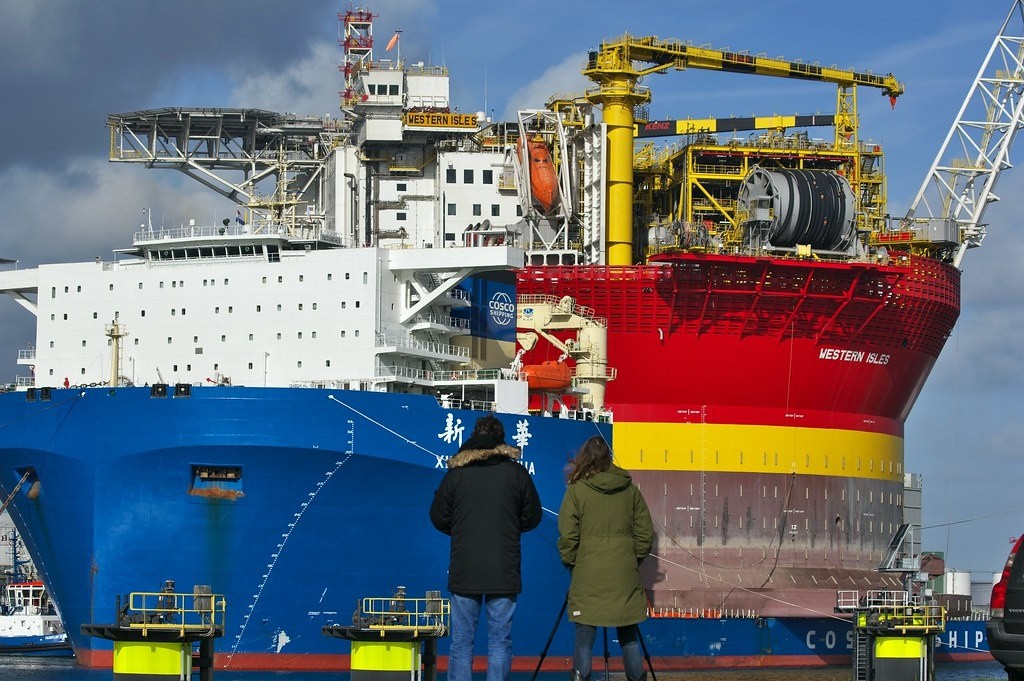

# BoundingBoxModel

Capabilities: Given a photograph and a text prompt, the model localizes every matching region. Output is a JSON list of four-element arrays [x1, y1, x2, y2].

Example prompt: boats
[[517, 360, 572, 390], [0, 582, 75, 658], [515, 133, 560, 212]]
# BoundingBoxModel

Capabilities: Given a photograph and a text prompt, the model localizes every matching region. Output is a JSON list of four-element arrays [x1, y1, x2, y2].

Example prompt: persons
[[557, 436, 655, 681], [429, 414, 543, 681]]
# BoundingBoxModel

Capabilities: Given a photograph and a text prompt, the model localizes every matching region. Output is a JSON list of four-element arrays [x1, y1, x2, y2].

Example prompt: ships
[[2, 6, 1024, 681]]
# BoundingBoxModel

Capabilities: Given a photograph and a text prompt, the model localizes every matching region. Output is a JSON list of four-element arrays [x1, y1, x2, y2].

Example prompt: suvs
[[985, 533, 1024, 681]]
